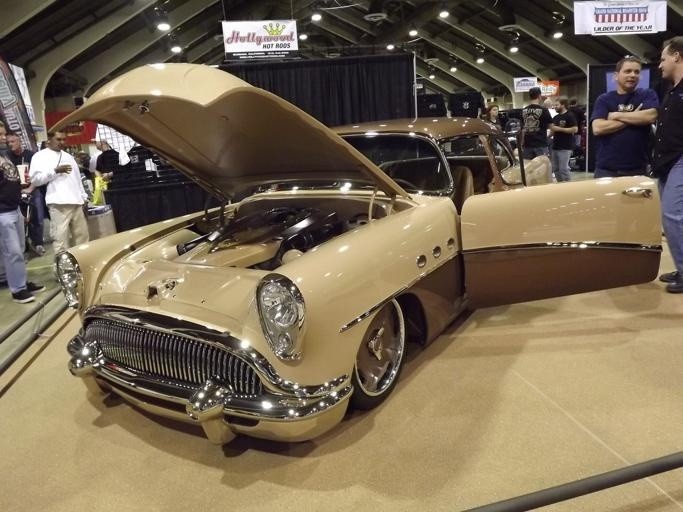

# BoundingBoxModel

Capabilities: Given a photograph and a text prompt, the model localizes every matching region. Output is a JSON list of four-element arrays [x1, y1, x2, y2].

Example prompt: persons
[[549, 98, 578, 182], [590, 57, 658, 178], [94, 139, 118, 191], [650, 36, 682, 292], [6, 132, 44, 265], [27, 130, 89, 252], [519, 87, 552, 160], [486, 104, 502, 132], [569, 97, 584, 148], [0, 120, 44, 303], [75, 148, 93, 201]]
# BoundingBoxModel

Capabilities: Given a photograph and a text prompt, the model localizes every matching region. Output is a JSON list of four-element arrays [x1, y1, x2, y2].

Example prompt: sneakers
[[668, 279, 683, 294], [660, 271, 683, 282], [26, 280, 46, 293], [12, 289, 35, 303], [36, 245, 46, 254]]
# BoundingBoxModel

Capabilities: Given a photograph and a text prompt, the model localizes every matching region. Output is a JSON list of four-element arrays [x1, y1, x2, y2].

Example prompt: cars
[[45, 62, 662, 446]]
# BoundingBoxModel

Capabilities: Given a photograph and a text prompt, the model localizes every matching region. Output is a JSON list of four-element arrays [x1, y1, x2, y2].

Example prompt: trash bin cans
[[86, 204, 118, 242]]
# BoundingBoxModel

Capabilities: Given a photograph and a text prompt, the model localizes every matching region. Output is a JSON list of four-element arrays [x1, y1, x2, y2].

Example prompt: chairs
[[445, 166, 475, 214]]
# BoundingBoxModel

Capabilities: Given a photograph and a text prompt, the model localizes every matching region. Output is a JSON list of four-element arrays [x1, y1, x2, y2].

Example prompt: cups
[[61, 162, 72, 174], [15, 164, 31, 186]]
